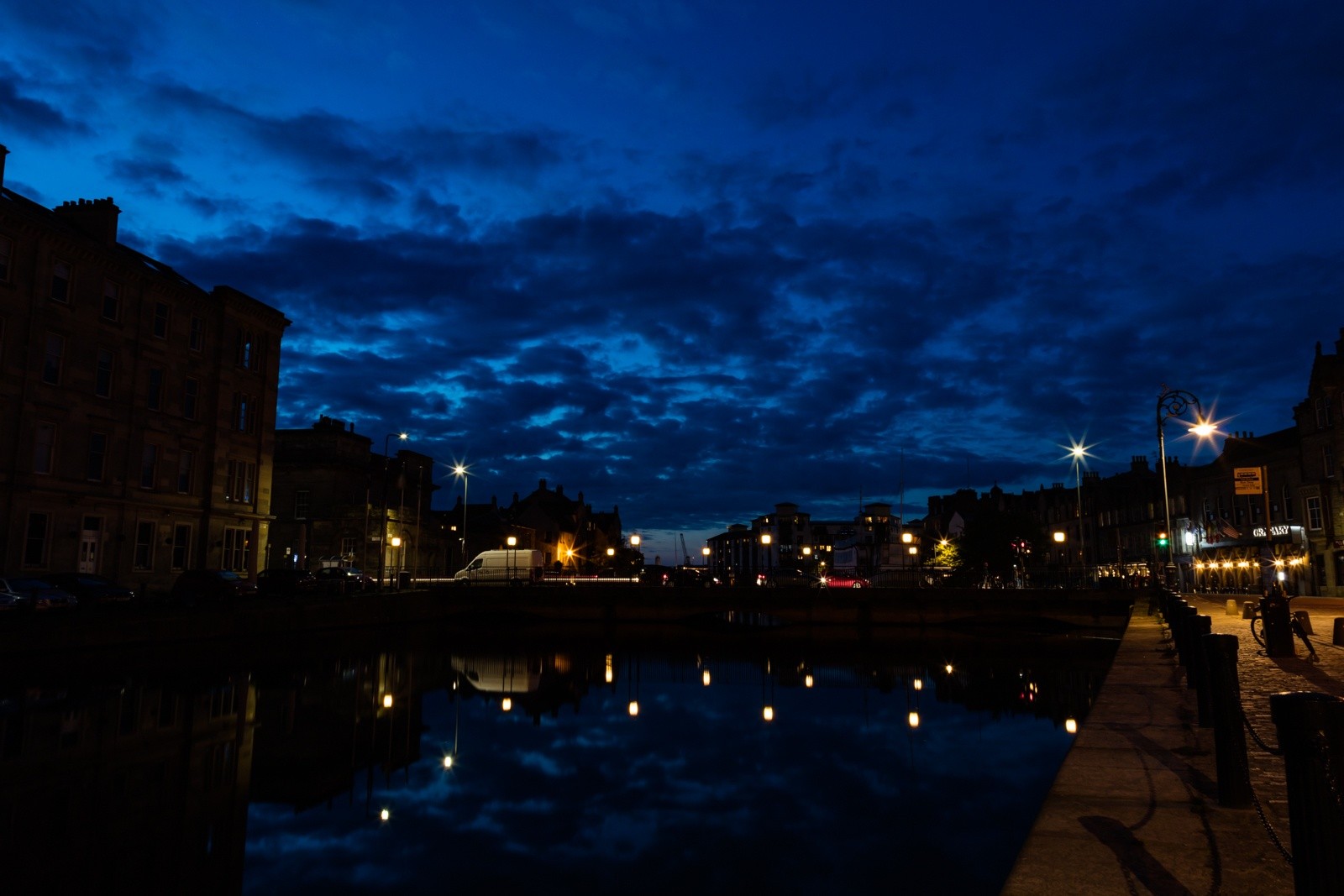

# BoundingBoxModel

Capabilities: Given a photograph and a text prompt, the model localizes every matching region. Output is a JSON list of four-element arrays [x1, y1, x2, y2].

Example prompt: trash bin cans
[[400, 571, 411, 589], [1259, 597, 1294, 656]]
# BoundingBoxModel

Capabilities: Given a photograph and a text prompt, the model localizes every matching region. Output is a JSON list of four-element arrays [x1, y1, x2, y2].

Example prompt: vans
[[454, 548, 545, 584]]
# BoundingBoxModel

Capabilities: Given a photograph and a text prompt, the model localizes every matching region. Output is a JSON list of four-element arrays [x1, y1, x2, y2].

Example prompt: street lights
[[454, 465, 468, 570], [384, 431, 408, 457], [1155, 380, 1219, 594], [1069, 442, 1091, 590]]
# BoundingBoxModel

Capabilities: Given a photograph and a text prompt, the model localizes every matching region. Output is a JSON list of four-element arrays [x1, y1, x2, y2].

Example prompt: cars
[[756, 568, 821, 588], [819, 568, 873, 590], [315, 566, 364, 592], [231, 568, 318, 600], [37, 572, 134, 604], [0, 578, 79, 608]]
